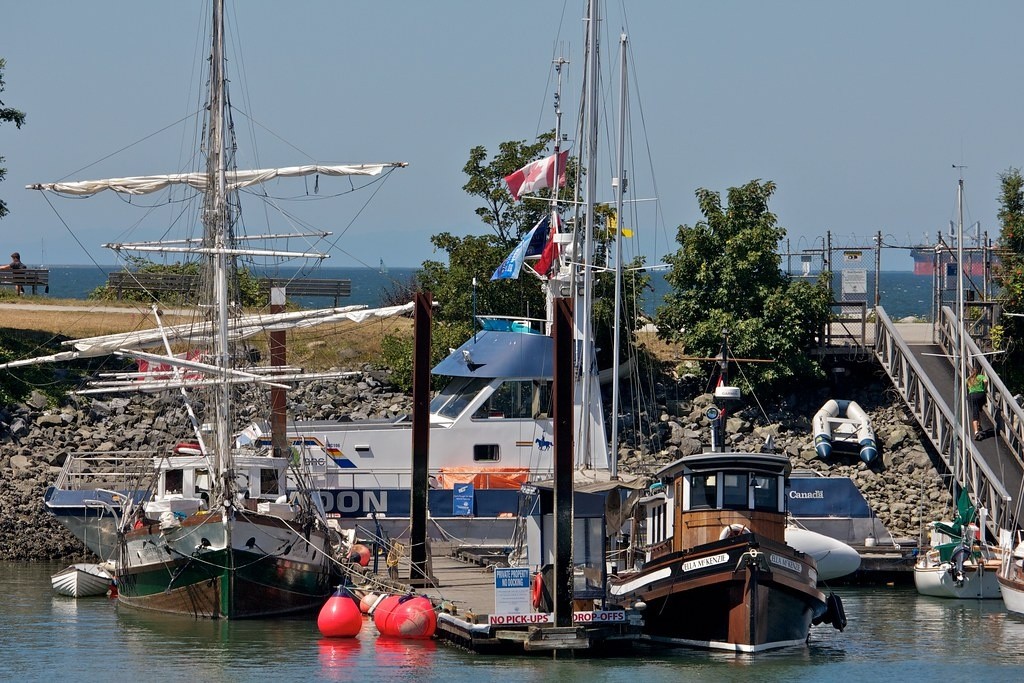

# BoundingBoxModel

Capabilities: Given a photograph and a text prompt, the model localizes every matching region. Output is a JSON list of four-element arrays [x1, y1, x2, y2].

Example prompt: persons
[[966, 368, 989, 440], [0, 252, 25, 296]]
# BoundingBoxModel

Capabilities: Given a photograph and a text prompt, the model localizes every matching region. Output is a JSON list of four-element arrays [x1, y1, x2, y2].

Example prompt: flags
[[717, 375, 728, 417], [136, 349, 207, 383], [491, 148, 635, 283]]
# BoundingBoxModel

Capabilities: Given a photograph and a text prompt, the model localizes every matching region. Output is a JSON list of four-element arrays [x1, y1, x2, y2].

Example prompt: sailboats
[[0, 0, 1024, 658]]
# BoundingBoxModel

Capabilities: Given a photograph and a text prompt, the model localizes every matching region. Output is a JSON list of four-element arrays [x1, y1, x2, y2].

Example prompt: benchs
[[106, 273, 351, 310], [0, 267, 50, 296]]
